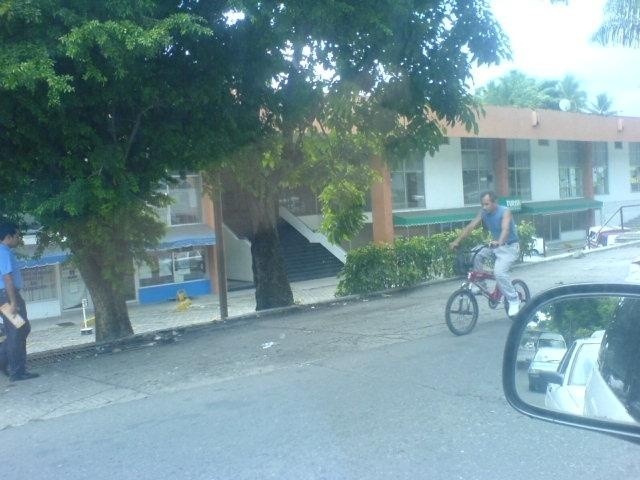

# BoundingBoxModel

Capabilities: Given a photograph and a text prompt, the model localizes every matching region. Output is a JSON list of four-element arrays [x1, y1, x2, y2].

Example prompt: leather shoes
[[14, 373, 39, 381]]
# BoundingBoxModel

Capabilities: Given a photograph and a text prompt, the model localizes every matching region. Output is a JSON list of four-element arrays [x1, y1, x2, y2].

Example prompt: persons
[[448, 189, 523, 317], [0, 221, 40, 381]]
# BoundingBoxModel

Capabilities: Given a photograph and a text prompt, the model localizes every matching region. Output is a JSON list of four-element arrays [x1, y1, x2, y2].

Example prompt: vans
[[586, 226, 629, 248]]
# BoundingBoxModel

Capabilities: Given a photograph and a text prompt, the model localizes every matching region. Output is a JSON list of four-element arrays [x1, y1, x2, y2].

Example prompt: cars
[[517, 330, 606, 417], [527, 237, 547, 256]]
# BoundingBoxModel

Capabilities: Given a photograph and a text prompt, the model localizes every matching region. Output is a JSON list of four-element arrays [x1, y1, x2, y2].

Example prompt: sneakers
[[508, 300, 521, 316], [472, 282, 487, 295]]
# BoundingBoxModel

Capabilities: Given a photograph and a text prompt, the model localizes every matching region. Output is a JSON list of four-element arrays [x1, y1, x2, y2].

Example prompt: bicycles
[[445, 243, 531, 335]]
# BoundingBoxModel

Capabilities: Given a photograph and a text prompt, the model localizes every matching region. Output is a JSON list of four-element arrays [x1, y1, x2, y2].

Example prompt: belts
[[491, 241, 515, 249]]
